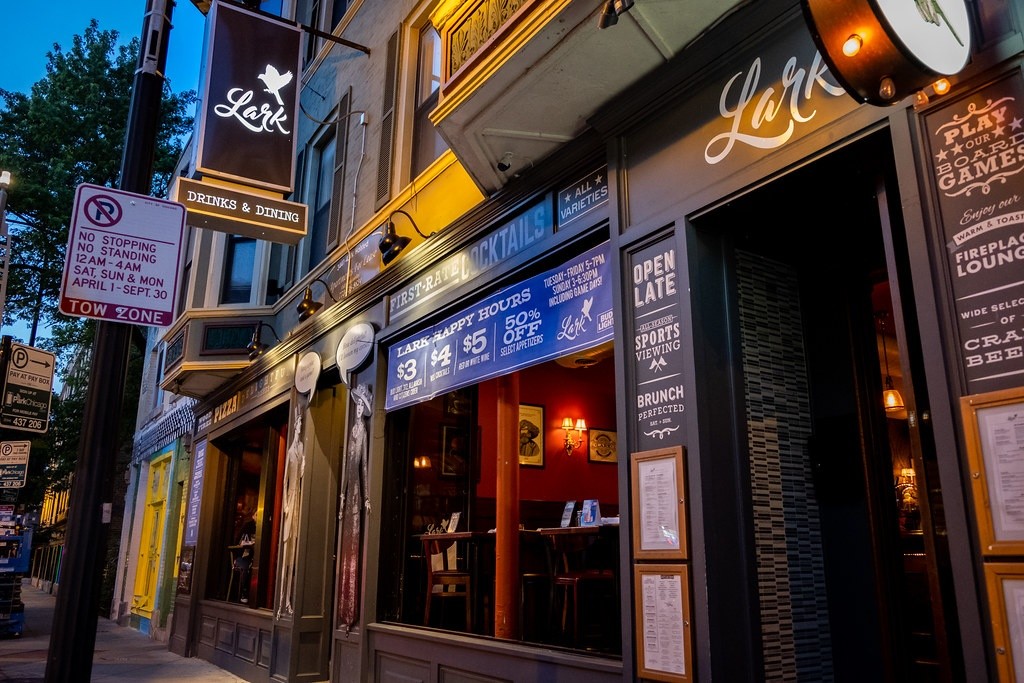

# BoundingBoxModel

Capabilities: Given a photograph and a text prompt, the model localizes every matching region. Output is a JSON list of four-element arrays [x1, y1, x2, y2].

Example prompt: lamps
[[880, 322, 905, 412], [378, 212, 436, 266], [412, 449, 431, 469], [596, 0, 636, 28], [295, 280, 341, 324], [247, 323, 283, 361], [901, 468, 916, 484], [562, 417, 588, 457]]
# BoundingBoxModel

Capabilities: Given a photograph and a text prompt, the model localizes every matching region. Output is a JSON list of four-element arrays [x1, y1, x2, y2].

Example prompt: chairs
[[543, 533, 614, 640], [424, 534, 473, 632]]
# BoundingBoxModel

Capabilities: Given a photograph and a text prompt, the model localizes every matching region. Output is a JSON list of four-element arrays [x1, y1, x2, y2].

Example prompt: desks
[[418, 529, 495, 636], [535, 524, 620, 637]]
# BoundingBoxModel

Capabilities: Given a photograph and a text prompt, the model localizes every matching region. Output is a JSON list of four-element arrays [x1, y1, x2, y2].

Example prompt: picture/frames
[[588, 428, 618, 463], [519, 403, 545, 469], [439, 423, 468, 480]]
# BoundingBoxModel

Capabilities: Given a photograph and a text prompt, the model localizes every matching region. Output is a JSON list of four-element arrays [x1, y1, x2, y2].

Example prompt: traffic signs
[[0, 343, 56, 434], [0, 441, 31, 488]]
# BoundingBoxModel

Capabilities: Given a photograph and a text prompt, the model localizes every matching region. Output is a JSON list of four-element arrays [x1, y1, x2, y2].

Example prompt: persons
[[236, 509, 257, 603]]
